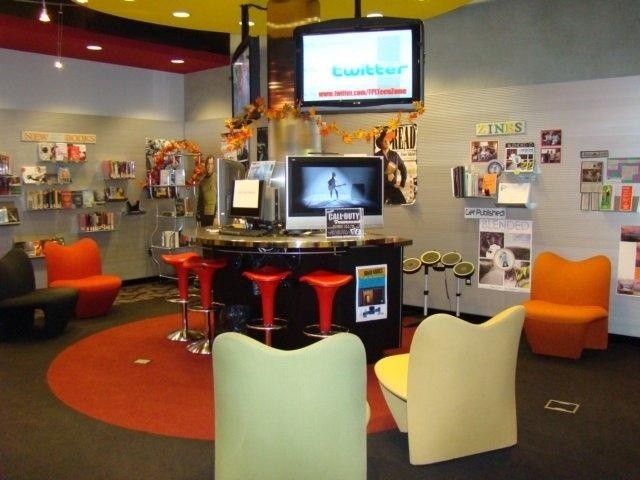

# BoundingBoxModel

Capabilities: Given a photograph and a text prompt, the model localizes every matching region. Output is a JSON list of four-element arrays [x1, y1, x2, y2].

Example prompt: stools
[[160, 251, 354, 355]]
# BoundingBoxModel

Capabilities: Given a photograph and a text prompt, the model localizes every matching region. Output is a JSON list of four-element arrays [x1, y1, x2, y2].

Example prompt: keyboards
[[219, 227, 267, 237]]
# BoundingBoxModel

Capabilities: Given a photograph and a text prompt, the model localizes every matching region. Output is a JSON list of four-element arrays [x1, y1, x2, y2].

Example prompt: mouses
[[277, 228, 287, 236]]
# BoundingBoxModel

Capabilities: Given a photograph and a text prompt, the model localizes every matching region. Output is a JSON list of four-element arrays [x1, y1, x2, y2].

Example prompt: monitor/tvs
[[294, 16, 424, 112], [214, 159, 245, 225], [229, 177, 266, 221], [232, 37, 260, 127], [285, 155, 385, 229]]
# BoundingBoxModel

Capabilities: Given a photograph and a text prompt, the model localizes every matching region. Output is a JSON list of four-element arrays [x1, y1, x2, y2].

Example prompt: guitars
[[328, 183, 346, 190]]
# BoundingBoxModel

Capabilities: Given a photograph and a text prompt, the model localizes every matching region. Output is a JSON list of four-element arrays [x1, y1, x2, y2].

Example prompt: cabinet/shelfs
[[1, 159, 147, 260], [143, 153, 201, 281]]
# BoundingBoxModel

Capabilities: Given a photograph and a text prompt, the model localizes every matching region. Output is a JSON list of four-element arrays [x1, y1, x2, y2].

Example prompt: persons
[[233, 54, 251, 116], [473, 143, 497, 162], [375, 130, 408, 206], [327, 172, 339, 199], [542, 131, 561, 162], [506, 141, 533, 171], [195, 155, 219, 227], [583, 161, 603, 183]]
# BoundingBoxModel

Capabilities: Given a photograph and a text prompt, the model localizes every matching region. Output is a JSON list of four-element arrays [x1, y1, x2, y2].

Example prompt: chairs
[[373, 304, 526, 467], [211, 331, 371, 480], [523, 250, 611, 360], [0, 248, 79, 343], [43, 235, 123, 319]]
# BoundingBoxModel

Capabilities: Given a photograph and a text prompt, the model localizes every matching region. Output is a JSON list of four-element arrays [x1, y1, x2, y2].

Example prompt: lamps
[[54, 3, 63, 69], [37, 0, 52, 22]]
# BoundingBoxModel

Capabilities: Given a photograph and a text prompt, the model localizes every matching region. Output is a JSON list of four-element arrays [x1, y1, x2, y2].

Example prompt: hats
[[376, 126, 394, 148]]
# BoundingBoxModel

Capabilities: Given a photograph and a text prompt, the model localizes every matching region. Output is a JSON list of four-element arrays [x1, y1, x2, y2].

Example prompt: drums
[[441, 252, 461, 268], [453, 262, 474, 279], [421, 251, 441, 266], [403, 258, 421, 274]]
